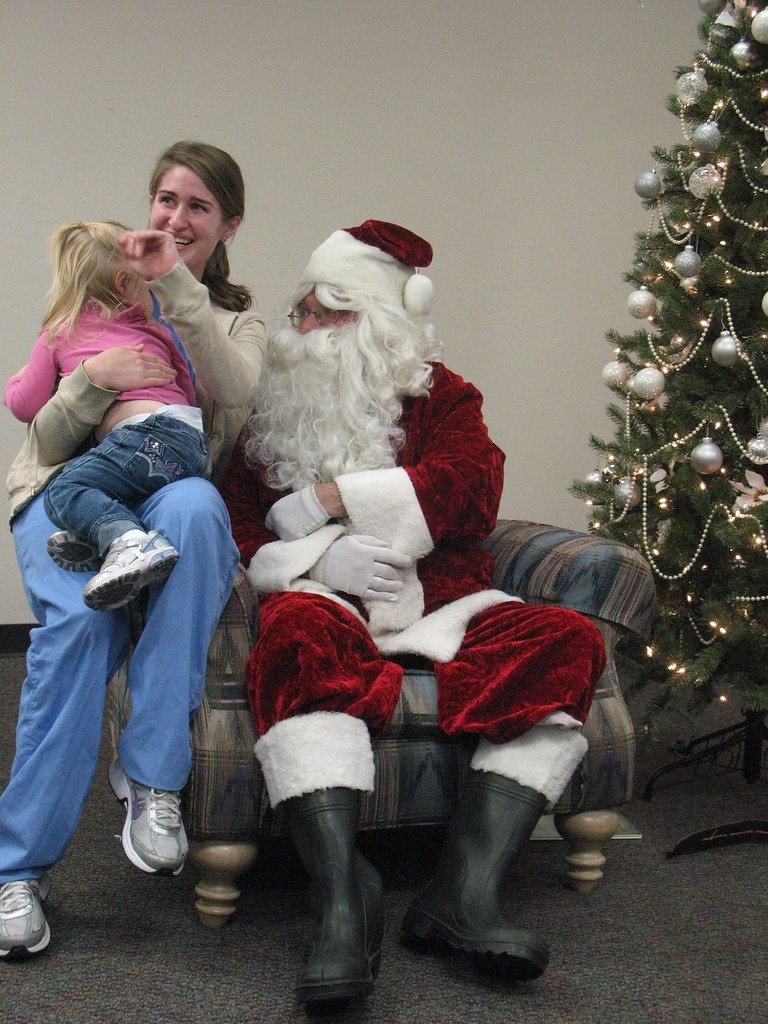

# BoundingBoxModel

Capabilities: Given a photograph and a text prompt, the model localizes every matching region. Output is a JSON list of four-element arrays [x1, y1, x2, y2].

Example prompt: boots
[[399, 728, 590, 980], [253, 712, 385, 1004]]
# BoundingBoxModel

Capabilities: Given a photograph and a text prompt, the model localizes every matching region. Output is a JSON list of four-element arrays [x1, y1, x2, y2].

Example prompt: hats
[[301, 219, 436, 319]]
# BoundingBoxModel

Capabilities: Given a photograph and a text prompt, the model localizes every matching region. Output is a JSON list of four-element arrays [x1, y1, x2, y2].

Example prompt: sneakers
[[107, 758, 189, 876], [46, 530, 106, 573], [82, 529, 181, 612], [0, 870, 51, 958]]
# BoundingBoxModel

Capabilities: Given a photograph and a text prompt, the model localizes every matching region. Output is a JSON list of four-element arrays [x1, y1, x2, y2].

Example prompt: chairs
[[101, 501, 657, 934]]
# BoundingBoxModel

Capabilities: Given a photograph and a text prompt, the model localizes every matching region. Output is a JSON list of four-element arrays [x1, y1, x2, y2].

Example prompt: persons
[[0, 142, 266, 964], [4, 221, 209, 613], [222, 220, 607, 1002]]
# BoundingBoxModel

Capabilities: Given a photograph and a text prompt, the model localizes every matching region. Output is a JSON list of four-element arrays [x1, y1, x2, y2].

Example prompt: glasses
[[287, 303, 351, 328]]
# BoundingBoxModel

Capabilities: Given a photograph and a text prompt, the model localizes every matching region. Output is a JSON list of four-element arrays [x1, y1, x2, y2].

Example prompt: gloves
[[309, 534, 413, 601], [264, 484, 331, 542]]
